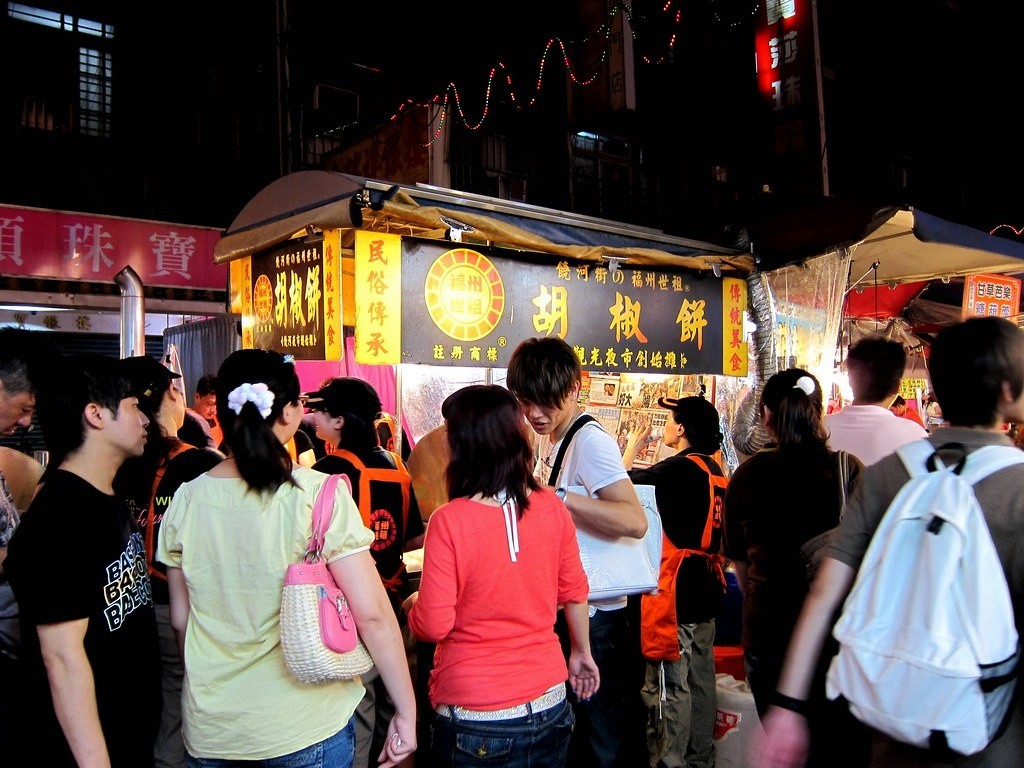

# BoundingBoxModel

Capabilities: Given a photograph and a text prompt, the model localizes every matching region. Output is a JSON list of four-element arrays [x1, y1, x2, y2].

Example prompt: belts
[[435, 683, 568, 722]]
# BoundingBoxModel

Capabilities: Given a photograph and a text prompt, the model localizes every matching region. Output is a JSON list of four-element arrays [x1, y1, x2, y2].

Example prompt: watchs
[[553, 488, 568, 503]]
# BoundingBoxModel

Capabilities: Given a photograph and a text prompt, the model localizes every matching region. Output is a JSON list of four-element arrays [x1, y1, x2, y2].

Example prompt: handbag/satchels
[[279, 473, 376, 683], [930, 416, 944, 425], [801, 449, 851, 585], [551, 485, 663, 600]]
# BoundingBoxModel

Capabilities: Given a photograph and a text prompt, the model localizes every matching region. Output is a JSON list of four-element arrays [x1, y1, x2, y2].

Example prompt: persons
[[0, 315, 1024, 768]]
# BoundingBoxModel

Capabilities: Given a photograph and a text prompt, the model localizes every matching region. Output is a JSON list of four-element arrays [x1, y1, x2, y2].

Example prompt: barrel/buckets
[[714, 672, 762, 768]]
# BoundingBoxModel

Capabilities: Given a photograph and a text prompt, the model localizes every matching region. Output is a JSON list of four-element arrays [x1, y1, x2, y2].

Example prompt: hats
[[658, 396, 720, 434], [302, 377, 382, 424], [119, 356, 182, 382]]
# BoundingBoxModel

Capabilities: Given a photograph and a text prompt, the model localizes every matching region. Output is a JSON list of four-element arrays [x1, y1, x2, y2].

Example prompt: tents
[[740, 203, 1024, 415]]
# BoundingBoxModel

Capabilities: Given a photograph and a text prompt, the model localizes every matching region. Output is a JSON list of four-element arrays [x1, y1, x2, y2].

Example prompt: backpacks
[[824, 439, 1024, 758]]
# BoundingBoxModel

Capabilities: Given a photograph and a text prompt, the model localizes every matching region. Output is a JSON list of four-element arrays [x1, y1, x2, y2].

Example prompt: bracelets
[[771, 689, 807, 716]]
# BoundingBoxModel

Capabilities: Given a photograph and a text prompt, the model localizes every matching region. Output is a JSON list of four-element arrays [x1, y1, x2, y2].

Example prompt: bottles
[[626, 427, 665, 445]]
